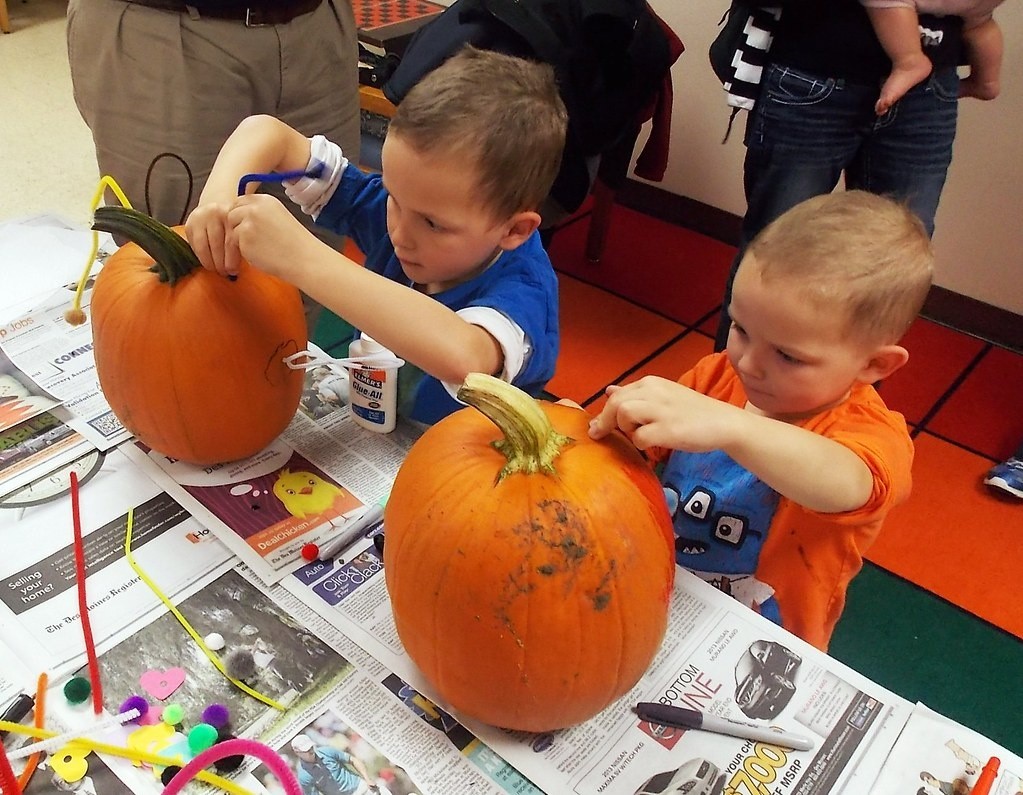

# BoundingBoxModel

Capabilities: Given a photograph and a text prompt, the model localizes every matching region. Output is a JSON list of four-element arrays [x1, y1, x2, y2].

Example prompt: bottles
[[348, 332, 398, 434]]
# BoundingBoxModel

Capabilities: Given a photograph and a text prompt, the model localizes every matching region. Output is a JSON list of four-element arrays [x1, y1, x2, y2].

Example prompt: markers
[[374, 531, 385, 552]]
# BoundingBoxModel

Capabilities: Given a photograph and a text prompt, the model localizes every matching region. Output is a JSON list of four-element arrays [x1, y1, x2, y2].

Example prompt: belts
[[119, 0, 322, 28]]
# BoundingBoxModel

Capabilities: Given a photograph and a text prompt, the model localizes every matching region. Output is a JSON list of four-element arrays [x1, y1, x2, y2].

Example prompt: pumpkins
[[381, 371, 674, 731], [90, 206, 308, 467]]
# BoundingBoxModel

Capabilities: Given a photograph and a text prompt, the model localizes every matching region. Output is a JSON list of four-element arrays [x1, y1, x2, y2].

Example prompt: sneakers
[[983, 455, 1023, 498]]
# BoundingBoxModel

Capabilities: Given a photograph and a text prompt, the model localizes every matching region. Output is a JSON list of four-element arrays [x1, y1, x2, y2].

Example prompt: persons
[[708, 0, 1004, 391], [982, 441, 1023, 502], [67, 0, 361, 345], [586, 189, 936, 654], [182, 43, 568, 431], [312, 368, 350, 405], [291, 733, 382, 795]]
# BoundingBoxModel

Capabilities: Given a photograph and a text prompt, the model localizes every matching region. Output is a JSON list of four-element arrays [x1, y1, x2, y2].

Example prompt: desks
[[0, 276, 1023, 795], [352, 0, 448, 41]]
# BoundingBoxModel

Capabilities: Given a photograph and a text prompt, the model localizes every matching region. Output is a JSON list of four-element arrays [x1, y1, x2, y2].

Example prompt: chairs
[[247, 0, 684, 265]]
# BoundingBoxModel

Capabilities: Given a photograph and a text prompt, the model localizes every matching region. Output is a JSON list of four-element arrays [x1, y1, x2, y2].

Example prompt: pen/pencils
[[0, 692, 35, 740], [636, 702, 814, 751], [319, 496, 388, 561]]
